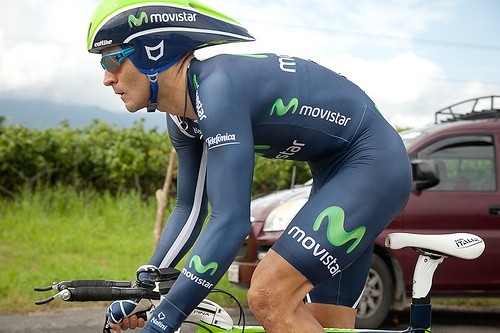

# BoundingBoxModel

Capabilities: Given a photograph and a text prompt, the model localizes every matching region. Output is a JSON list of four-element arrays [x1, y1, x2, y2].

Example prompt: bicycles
[[30, 233, 485, 333]]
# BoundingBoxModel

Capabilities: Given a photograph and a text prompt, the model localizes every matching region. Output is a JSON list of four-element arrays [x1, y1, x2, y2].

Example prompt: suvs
[[229, 96, 500, 328]]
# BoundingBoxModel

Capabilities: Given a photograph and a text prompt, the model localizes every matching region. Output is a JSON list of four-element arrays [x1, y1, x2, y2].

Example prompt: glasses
[[99, 45, 135, 74]]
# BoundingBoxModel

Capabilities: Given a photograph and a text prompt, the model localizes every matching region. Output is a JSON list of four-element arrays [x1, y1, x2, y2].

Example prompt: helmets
[[87, 0, 257, 73]]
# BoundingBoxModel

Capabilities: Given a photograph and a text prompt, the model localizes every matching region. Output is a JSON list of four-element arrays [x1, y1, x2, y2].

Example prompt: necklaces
[[177, 83, 191, 132]]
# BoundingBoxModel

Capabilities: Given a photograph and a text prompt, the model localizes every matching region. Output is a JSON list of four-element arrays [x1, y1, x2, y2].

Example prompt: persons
[[85, 0, 413, 333]]
